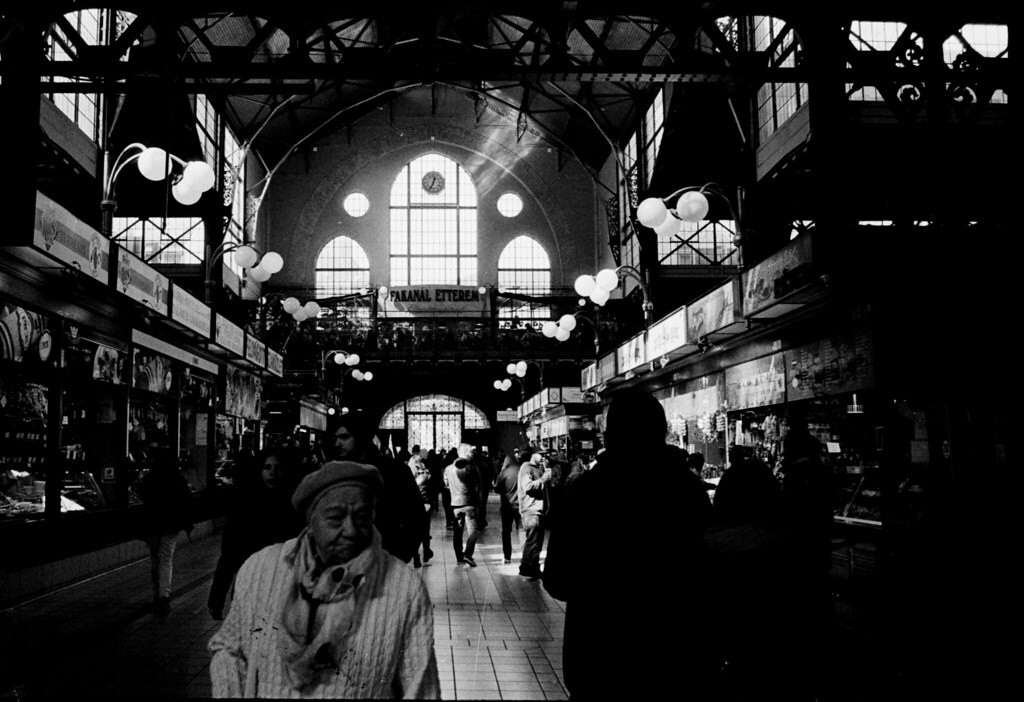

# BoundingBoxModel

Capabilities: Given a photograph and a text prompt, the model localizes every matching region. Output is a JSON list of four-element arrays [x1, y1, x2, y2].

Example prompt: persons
[[85, 386, 837, 702]]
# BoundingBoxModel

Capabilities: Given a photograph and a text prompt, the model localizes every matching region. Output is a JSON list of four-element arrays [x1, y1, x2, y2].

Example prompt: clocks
[[423, 172, 444, 192]]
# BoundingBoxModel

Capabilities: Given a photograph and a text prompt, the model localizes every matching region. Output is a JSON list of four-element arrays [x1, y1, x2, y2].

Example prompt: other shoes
[[521, 565, 543, 580], [457, 558, 461, 563], [423, 550, 432, 561], [464, 555, 476, 568], [414, 556, 421, 567]]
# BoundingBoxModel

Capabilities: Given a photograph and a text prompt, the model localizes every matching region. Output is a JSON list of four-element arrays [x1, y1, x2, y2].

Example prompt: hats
[[292, 459, 384, 523]]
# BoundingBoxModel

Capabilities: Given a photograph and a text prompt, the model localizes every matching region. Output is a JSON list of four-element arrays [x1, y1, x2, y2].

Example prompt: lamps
[[342, 369, 373, 382], [105, 142, 215, 206], [507, 358, 541, 378], [543, 311, 600, 358], [574, 267, 661, 311], [261, 293, 318, 322], [209, 241, 285, 287], [324, 349, 361, 368], [635, 180, 737, 239], [495, 377, 525, 400]]
